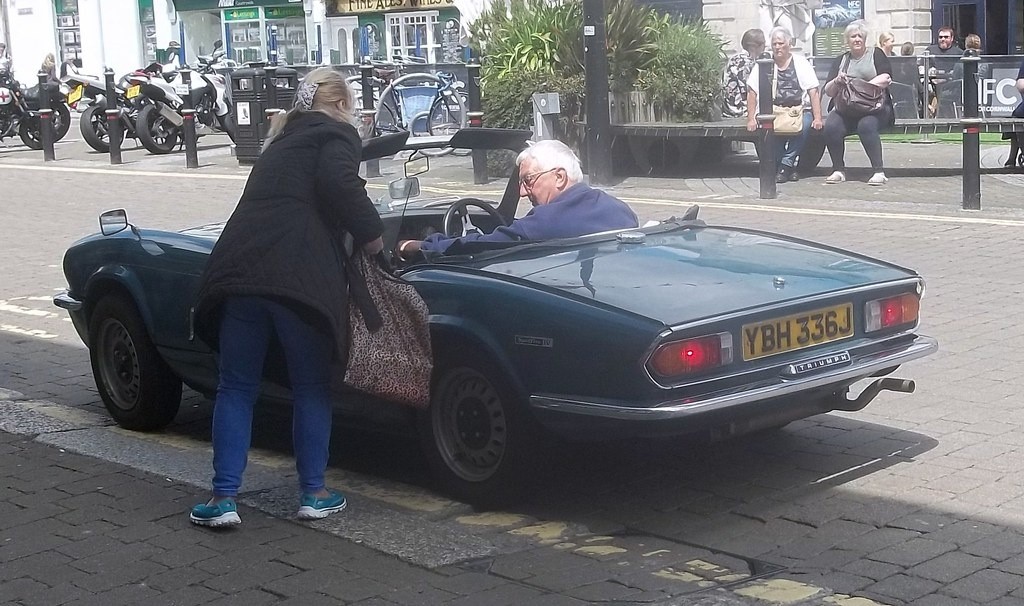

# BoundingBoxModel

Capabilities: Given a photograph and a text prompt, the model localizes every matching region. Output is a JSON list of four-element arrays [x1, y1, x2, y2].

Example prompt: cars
[[52, 128, 937, 512]]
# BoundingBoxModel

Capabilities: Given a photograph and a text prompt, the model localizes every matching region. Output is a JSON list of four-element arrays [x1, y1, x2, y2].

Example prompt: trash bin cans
[[230, 62, 299, 167]]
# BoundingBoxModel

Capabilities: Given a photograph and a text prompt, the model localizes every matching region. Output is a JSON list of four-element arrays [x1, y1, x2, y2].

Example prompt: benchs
[[576, 114, 760, 177]]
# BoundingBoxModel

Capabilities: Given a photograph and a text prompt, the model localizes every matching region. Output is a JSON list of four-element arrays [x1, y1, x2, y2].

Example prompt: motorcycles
[[0, 57, 71, 150], [59, 40, 243, 155]]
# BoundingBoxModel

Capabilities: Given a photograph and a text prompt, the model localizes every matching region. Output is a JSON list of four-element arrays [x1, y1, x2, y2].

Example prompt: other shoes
[[786, 167, 799, 181], [826, 171, 846, 182], [775, 165, 788, 182], [868, 171, 887, 185]]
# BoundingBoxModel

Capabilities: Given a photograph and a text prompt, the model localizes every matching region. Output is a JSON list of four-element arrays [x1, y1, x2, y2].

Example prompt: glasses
[[938, 36, 952, 38], [517, 166, 556, 189]]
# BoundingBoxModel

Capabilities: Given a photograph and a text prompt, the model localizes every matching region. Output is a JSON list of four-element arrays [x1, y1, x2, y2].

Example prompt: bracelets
[[833, 78, 839, 87], [400, 240, 415, 253]]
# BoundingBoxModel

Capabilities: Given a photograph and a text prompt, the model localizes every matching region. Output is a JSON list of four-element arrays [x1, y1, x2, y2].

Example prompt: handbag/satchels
[[832, 77, 886, 117], [772, 105, 804, 136], [341, 244, 432, 411]]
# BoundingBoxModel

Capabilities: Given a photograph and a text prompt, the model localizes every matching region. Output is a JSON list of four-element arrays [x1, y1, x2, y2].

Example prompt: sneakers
[[188, 496, 241, 527], [298, 488, 346, 519]]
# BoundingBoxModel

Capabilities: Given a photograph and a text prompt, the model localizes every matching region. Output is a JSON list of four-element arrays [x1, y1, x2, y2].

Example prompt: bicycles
[[351, 68, 474, 157]]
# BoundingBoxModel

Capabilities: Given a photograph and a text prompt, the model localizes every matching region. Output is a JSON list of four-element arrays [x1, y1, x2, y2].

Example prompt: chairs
[[805, 50, 1024, 137]]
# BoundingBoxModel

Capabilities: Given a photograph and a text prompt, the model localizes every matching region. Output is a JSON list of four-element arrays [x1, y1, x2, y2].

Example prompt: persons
[[400, 138, 640, 258], [721, 27, 822, 183], [0, 43, 12, 73], [822, 21, 891, 185], [41, 54, 61, 88], [188, 63, 391, 528], [1002, 59, 1024, 167], [878, 33, 938, 125], [920, 26, 982, 121]]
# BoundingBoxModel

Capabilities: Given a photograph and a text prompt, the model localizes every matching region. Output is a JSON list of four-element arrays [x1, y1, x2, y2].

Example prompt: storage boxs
[[63, 52, 76, 60], [61, 16, 74, 27], [75, 15, 80, 26], [77, 52, 82, 59], [69, 47, 75, 53], [76, 32, 81, 42], [63, 31, 75, 43]]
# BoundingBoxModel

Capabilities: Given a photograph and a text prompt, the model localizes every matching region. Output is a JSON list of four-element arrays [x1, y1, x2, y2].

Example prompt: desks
[[919, 74, 952, 94]]
